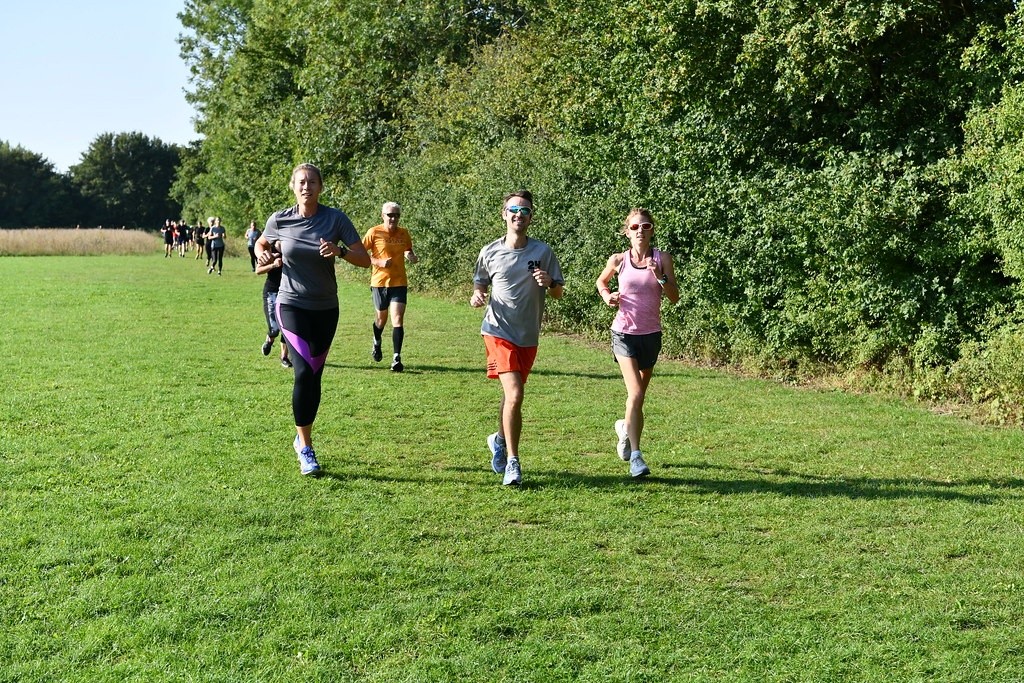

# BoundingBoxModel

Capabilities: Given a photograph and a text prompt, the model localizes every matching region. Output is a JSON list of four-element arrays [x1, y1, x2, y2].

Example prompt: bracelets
[[599, 287, 609, 294]]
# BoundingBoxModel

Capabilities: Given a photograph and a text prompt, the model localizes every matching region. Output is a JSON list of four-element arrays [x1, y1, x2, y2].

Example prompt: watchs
[[338, 245, 347, 259], [547, 280, 558, 291], [659, 274, 667, 288]]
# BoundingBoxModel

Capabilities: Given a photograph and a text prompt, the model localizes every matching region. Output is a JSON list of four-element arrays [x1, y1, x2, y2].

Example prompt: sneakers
[[630, 455, 650, 477], [390, 362, 403, 371], [297, 447, 320, 475], [280, 358, 293, 367], [292, 435, 301, 453], [372, 336, 382, 362], [615, 419, 631, 461], [487, 431, 507, 474], [262, 334, 274, 355], [503, 460, 522, 485]]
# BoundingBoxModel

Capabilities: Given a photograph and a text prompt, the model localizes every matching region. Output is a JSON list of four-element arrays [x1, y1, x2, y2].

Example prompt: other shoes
[[164, 247, 255, 275]]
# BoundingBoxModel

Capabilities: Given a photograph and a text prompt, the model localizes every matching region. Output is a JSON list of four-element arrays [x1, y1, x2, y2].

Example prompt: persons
[[245, 221, 263, 270], [255, 241, 293, 368], [362, 202, 418, 371], [193, 221, 205, 259], [470, 193, 564, 487], [255, 163, 371, 476], [203, 217, 226, 277], [161, 218, 194, 258], [596, 208, 680, 479]]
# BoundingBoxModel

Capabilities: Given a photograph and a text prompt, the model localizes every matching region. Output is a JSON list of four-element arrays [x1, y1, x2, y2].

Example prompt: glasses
[[384, 212, 400, 218], [627, 222, 654, 229], [505, 205, 532, 215]]
